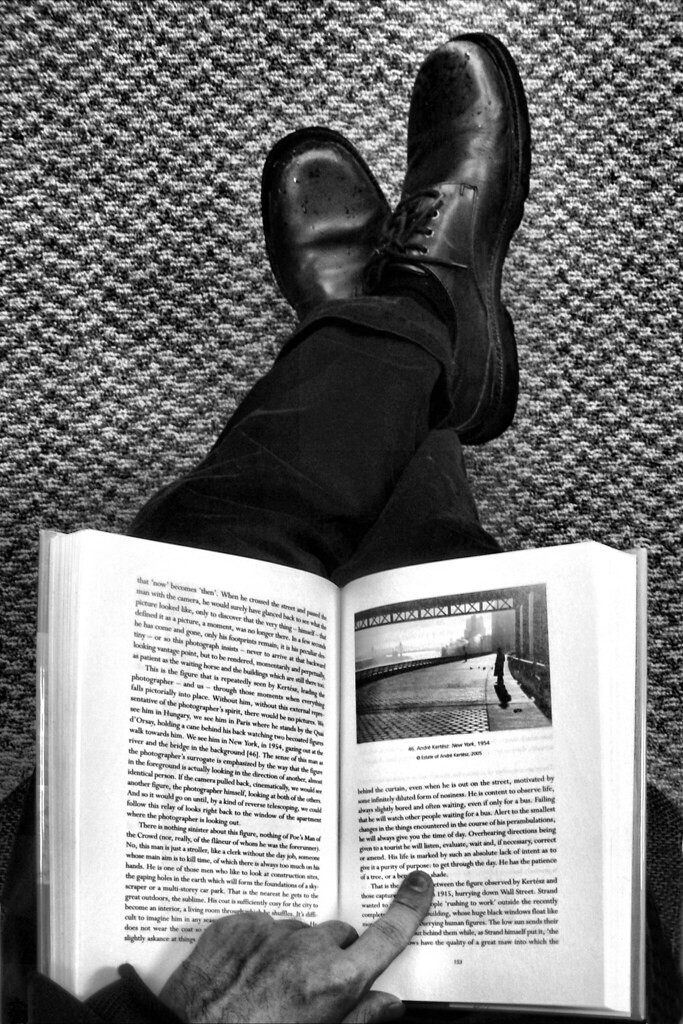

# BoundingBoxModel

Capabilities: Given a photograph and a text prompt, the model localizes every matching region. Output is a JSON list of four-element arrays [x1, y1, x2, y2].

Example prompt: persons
[[0, 32, 683, 1024]]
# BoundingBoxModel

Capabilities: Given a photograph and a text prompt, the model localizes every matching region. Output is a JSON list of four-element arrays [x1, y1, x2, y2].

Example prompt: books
[[36, 528, 646, 1020]]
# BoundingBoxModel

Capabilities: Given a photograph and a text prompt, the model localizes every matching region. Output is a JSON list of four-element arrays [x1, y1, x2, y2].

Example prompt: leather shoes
[[365, 33, 532, 446], [261, 126, 392, 309]]
